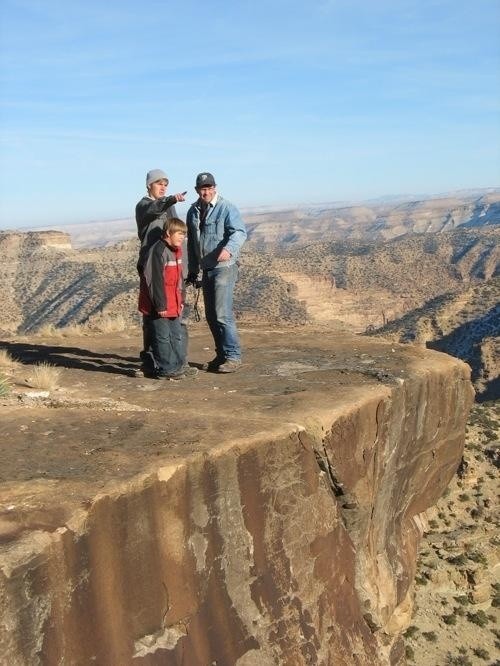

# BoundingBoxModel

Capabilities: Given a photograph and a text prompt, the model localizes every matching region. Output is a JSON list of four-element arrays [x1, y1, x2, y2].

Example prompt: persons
[[138, 218, 198, 379], [184, 173, 247, 373], [135, 169, 187, 378]]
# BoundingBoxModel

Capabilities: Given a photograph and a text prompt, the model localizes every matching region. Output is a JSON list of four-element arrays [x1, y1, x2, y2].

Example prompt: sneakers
[[218, 358, 242, 373], [156, 366, 199, 381], [201, 355, 226, 371], [133, 363, 154, 378]]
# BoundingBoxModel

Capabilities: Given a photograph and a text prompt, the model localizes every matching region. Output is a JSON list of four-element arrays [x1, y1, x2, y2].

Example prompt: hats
[[196, 172, 217, 189], [146, 168, 170, 188]]
